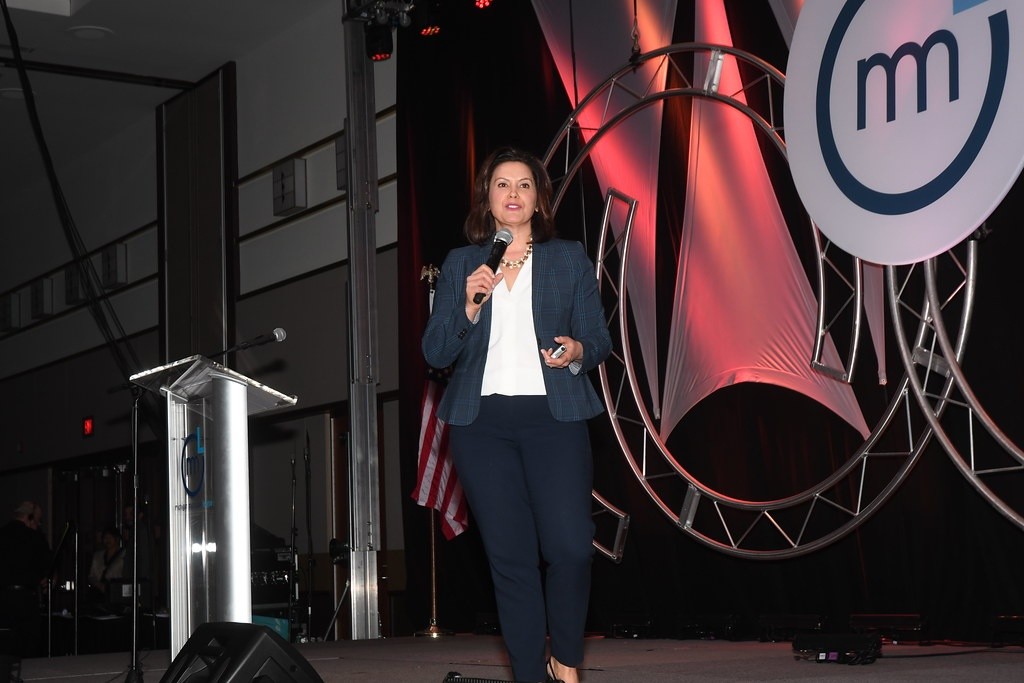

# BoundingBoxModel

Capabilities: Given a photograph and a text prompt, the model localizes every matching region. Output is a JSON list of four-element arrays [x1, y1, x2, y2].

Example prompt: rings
[[550, 365, 552, 368]]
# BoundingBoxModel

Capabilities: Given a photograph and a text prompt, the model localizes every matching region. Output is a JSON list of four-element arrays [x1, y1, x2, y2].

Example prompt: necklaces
[[499, 232, 532, 270]]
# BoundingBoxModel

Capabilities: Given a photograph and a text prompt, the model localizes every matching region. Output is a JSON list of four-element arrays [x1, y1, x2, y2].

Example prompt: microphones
[[227, 328, 287, 354], [472, 228, 514, 304]]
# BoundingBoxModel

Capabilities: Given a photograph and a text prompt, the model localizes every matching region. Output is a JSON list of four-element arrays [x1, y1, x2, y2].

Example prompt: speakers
[[158, 622, 324, 683]]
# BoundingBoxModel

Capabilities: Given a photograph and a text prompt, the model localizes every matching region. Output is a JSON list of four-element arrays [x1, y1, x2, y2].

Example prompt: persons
[[422, 146, 613, 683]]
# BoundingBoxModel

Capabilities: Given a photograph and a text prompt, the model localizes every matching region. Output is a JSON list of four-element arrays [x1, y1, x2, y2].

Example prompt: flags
[[411, 291, 469, 541]]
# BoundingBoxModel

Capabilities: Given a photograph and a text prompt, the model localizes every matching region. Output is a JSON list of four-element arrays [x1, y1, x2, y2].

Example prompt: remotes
[[551, 345, 566, 359]]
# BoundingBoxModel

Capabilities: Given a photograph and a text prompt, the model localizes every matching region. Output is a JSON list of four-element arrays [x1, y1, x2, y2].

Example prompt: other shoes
[[546, 656, 581, 683]]
